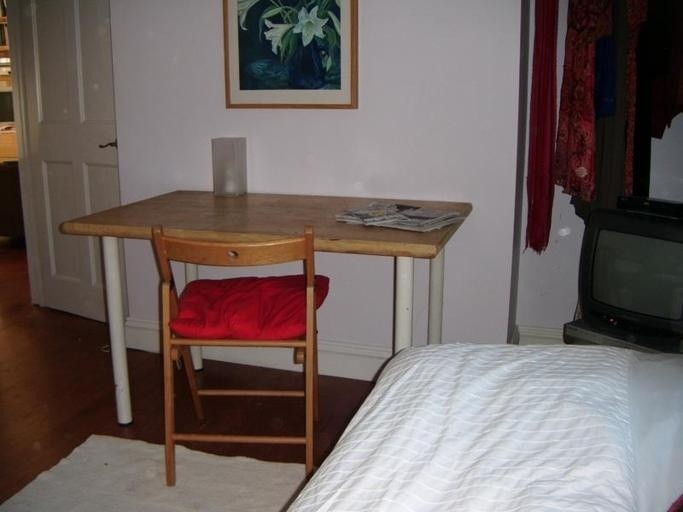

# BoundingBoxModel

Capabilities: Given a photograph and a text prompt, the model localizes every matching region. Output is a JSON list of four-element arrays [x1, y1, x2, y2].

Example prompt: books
[[336, 201, 460, 233]]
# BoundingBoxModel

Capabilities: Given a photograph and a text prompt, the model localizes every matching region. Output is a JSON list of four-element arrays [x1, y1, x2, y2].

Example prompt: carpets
[[0, 434, 315, 512]]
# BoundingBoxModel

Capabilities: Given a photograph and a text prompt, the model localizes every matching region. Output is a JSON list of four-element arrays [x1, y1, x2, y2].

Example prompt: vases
[[302, 44, 325, 89]]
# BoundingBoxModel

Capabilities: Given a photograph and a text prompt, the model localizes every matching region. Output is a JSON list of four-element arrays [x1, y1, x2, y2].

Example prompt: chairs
[[151, 224, 330, 488]]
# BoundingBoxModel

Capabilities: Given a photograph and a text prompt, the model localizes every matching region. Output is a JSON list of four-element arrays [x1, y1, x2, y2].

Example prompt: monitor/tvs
[[578, 198, 683, 350]]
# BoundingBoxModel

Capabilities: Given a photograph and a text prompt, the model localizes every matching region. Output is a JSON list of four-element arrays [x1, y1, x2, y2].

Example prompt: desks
[[59, 191, 473, 426]]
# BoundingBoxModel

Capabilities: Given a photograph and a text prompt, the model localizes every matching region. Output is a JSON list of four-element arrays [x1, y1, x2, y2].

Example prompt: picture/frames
[[223, 0, 359, 109]]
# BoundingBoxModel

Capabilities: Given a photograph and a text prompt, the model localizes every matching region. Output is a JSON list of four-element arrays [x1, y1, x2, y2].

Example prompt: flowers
[[238, 0, 340, 72]]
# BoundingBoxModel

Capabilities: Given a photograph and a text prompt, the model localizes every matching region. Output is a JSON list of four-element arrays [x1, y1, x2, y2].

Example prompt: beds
[[283, 343, 683, 512]]
